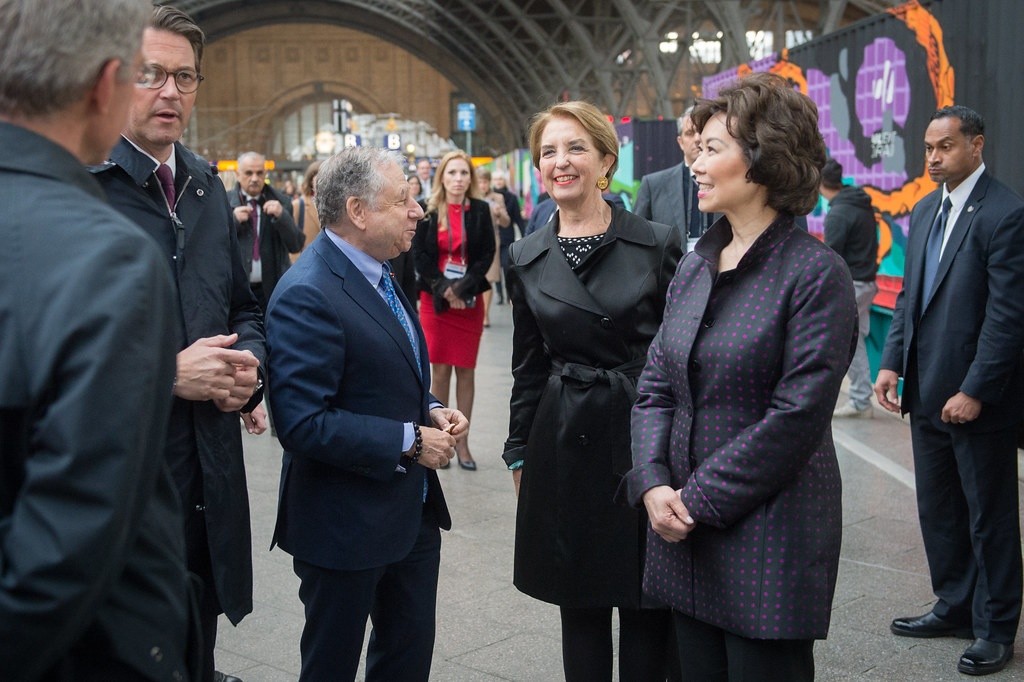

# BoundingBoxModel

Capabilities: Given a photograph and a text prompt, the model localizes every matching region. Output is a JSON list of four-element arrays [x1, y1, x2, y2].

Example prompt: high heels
[[454, 446, 476, 471]]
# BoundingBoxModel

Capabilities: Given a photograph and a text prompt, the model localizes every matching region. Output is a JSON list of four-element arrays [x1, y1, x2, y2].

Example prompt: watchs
[[254, 367, 263, 394]]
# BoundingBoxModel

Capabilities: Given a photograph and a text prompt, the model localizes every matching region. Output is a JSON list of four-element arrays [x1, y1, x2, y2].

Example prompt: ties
[[250, 198, 259, 261], [155, 164, 175, 212], [919, 196, 953, 321], [380, 267, 423, 380]]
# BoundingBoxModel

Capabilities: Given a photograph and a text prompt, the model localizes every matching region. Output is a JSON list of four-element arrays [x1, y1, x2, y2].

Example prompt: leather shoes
[[890, 611, 975, 640], [957, 637, 1014, 675]]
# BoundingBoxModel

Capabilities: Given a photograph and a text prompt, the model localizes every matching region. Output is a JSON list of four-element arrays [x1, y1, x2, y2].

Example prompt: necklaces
[[450, 204, 461, 213]]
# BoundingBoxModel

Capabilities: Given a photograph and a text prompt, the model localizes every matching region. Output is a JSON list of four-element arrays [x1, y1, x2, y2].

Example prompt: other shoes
[[484, 325, 490, 328], [833, 399, 874, 419]]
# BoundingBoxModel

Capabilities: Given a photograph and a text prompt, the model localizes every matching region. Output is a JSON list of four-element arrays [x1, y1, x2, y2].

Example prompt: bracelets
[[401, 420, 423, 468]]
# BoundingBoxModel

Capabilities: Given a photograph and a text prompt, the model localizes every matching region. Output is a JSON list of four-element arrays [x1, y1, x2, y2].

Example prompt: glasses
[[241, 194, 268, 216], [147, 63, 204, 94], [128, 60, 156, 88]]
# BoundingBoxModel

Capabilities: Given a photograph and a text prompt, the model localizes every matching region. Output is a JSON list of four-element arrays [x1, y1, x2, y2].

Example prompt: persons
[[266, 144, 469, 682], [226, 150, 627, 438], [818, 159, 879, 420], [0, 0, 178, 682], [501, 101, 682, 682], [613, 71, 860, 682], [412, 148, 497, 470], [89, 2, 267, 682], [631, 105, 725, 254], [870, 103, 1024, 675]]
